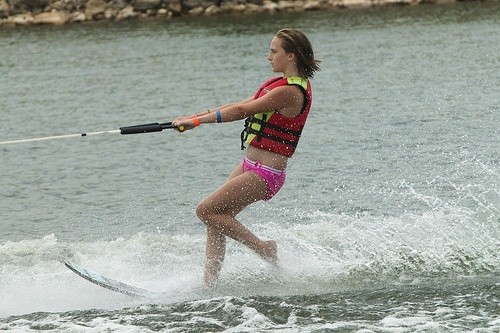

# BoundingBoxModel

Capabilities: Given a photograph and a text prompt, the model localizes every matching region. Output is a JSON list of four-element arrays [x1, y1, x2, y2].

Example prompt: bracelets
[[216, 111, 222, 124], [208, 109, 211, 113], [193, 117, 200, 126]]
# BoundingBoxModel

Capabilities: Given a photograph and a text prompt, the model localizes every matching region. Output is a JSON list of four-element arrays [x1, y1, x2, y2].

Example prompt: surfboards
[[65, 260, 160, 299]]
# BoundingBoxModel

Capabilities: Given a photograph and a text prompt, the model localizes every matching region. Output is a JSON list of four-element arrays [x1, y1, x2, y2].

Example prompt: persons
[[170, 27, 323, 294]]
